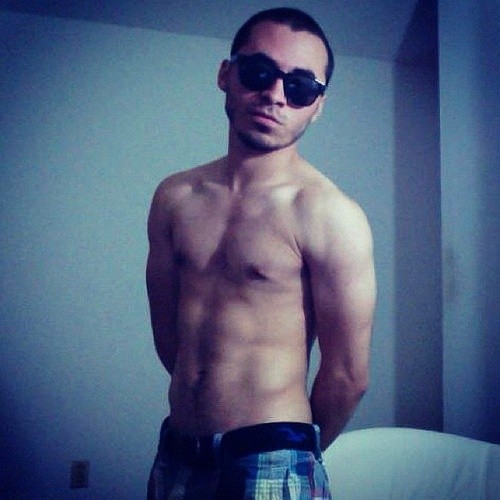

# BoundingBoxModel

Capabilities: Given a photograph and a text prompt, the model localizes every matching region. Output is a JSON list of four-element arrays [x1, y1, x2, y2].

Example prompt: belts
[[161, 422, 317, 465]]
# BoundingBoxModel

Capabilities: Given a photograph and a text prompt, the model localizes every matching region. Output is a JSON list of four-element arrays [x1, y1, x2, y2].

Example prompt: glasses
[[231, 52, 327, 107]]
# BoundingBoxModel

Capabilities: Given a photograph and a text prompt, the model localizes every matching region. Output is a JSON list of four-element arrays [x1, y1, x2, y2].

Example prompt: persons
[[146, 7, 376, 500]]
